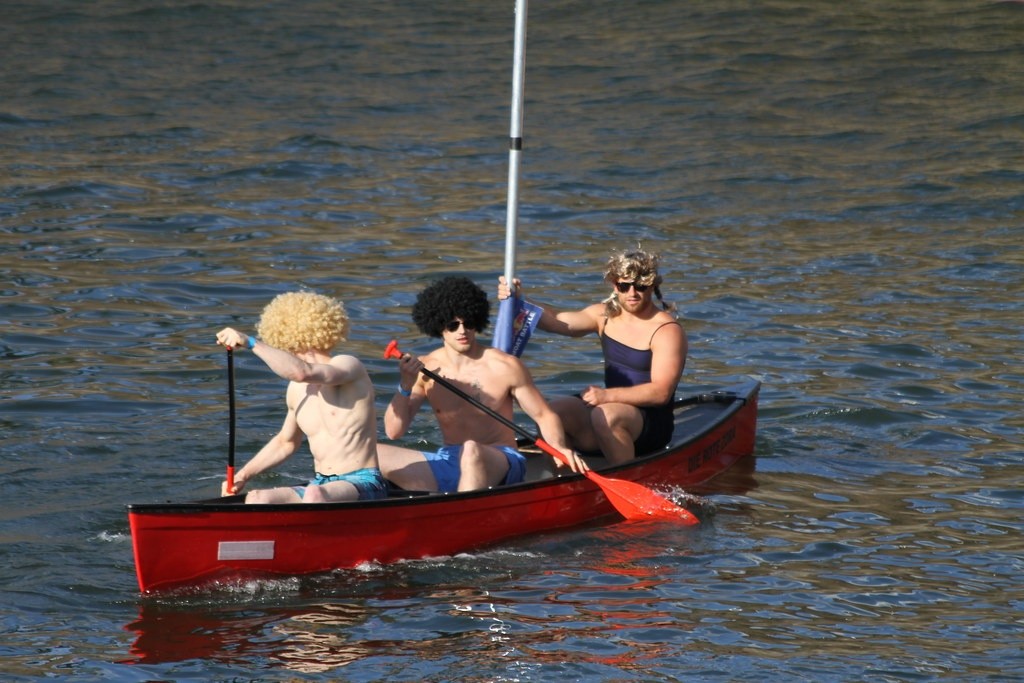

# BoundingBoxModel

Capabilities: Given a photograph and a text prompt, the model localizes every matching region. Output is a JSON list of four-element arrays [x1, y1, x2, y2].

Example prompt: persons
[[498, 249, 689, 467], [221, 289, 386, 505], [376, 276, 591, 493]]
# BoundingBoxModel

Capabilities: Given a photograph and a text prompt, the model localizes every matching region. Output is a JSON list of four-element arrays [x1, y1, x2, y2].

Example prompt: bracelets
[[398, 384, 411, 397], [246, 336, 255, 349], [519, 294, 525, 300]]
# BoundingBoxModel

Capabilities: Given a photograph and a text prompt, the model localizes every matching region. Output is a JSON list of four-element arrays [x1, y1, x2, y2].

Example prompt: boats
[[125, 382, 760, 591]]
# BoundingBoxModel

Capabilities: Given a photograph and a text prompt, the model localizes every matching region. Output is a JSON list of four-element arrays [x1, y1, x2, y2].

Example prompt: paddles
[[384, 340, 699, 524], [227, 345, 236, 493]]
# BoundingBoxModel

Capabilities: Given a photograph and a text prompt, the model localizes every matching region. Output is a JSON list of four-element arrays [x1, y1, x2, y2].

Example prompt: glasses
[[446, 322, 475, 333], [616, 281, 649, 293]]
